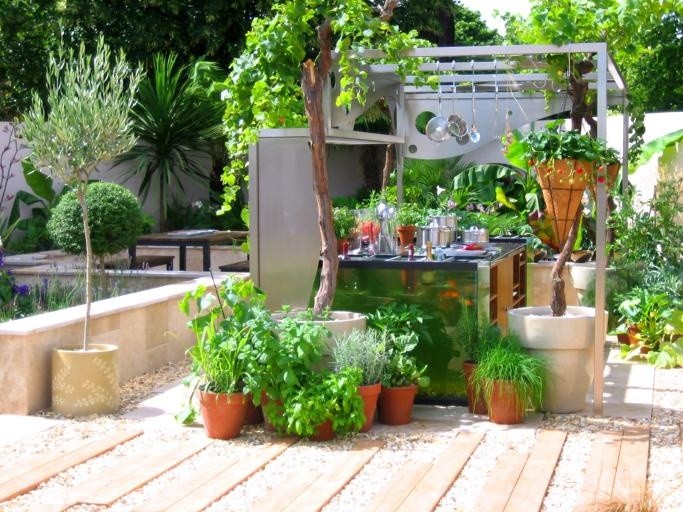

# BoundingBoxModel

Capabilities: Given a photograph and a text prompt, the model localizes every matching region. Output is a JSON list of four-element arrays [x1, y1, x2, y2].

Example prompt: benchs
[[217, 260, 249, 272], [94, 255, 176, 271]]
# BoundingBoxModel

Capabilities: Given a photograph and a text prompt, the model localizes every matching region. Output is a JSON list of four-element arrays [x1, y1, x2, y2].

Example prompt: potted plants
[[13, 28, 147, 417], [367, 302, 430, 424], [468, 334, 544, 425], [507, 0, 681, 416], [179, 275, 379, 441], [457, 307, 511, 415]]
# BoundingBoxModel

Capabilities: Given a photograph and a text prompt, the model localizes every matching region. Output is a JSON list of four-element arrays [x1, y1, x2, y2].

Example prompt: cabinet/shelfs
[[489, 245, 527, 340]]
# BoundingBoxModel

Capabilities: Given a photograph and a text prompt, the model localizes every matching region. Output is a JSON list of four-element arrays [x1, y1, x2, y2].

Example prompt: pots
[[416, 214, 489, 247]]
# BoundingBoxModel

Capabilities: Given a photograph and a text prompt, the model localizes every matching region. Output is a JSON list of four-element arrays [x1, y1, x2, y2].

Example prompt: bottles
[[426, 242, 433, 260], [408, 245, 414, 260]]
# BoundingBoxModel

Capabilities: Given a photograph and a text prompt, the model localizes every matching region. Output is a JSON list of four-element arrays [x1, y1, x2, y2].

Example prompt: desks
[[128, 228, 249, 271]]
